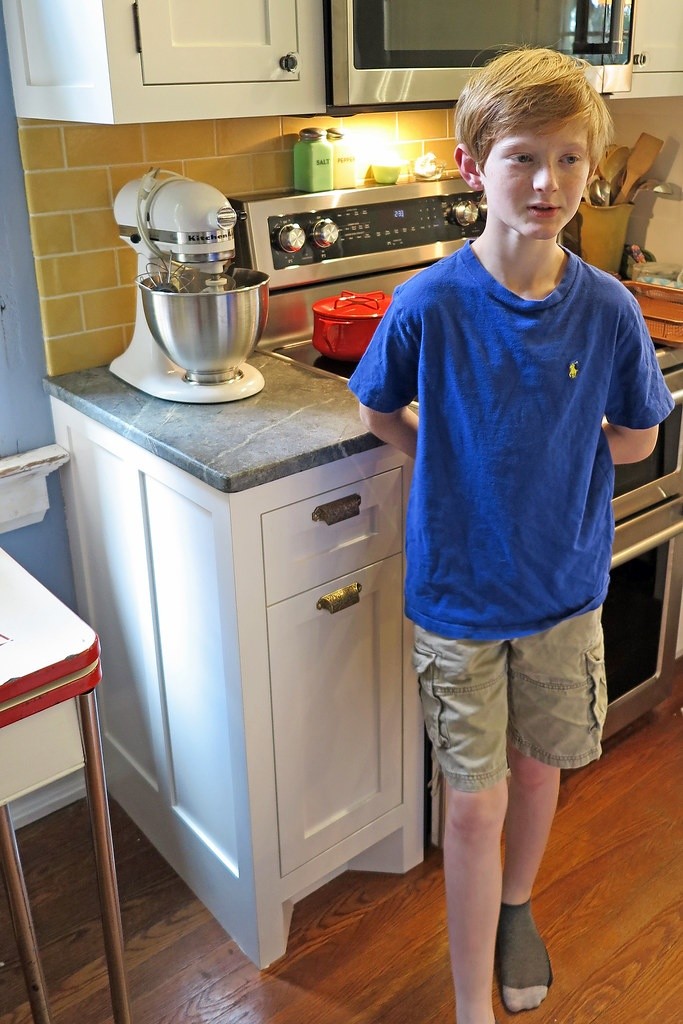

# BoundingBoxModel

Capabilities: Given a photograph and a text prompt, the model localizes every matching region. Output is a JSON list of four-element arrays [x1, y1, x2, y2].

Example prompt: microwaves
[[329, 0, 635, 106]]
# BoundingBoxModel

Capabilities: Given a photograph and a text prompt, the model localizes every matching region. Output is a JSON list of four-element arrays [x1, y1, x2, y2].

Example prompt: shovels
[[612, 132, 665, 205]]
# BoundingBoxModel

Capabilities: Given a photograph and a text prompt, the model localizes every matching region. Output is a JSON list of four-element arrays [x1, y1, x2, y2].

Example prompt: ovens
[[596, 369, 683, 746]]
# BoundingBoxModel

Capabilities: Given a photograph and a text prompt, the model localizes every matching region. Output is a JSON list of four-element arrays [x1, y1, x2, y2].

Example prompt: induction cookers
[[230, 176, 588, 407]]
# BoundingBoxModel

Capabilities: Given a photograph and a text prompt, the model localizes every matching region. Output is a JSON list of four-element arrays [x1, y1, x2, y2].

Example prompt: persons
[[345, 50, 675, 1024]]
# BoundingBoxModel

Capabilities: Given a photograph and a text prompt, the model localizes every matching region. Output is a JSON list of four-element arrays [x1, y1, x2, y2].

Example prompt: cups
[[575, 202, 635, 278]]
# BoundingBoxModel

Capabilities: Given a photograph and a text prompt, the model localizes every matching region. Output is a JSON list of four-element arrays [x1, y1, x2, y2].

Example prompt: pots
[[312, 289, 393, 363]]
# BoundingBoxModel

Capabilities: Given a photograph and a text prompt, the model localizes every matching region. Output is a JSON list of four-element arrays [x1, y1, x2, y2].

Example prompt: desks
[[0, 543, 131, 1024]]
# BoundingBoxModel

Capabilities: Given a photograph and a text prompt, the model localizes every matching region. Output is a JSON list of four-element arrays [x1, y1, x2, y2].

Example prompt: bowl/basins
[[411, 161, 446, 181], [138, 268, 271, 382]]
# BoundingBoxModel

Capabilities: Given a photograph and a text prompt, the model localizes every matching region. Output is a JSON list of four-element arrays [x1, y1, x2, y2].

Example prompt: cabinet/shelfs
[[48, 396, 429, 971], [0, 0, 331, 127], [603, 1, 683, 98]]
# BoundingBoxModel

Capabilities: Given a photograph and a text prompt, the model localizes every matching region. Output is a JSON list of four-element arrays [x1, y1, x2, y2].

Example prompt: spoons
[[604, 146, 631, 206]]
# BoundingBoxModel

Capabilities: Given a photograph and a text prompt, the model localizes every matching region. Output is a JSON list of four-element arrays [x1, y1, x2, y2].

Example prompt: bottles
[[326, 127, 357, 190], [293, 128, 333, 192]]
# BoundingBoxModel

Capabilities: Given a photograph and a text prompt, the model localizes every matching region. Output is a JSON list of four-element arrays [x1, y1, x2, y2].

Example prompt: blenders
[[108, 167, 271, 405]]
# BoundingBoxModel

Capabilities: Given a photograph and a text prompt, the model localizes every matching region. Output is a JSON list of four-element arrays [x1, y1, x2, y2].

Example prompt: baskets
[[620, 281, 683, 347]]
